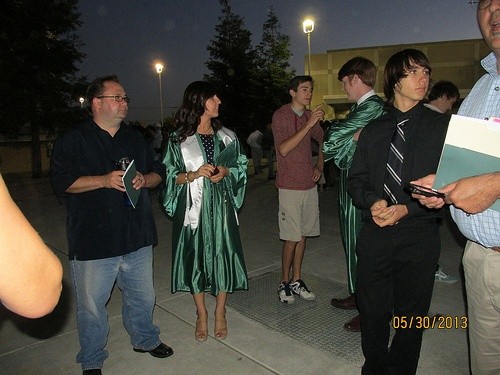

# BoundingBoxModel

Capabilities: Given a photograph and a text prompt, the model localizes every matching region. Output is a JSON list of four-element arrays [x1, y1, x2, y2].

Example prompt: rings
[[138, 178, 142, 183]]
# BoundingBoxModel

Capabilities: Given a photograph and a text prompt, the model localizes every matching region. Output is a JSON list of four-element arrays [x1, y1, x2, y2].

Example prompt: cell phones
[[404, 183, 447, 200], [211, 166, 219, 177]]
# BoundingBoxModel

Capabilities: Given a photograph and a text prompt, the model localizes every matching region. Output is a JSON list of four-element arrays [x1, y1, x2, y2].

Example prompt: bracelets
[[161, 79, 249, 342], [184, 170, 195, 183]]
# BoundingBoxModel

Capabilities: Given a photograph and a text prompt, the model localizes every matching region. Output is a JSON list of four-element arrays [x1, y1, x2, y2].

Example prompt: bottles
[[119, 156, 132, 206]]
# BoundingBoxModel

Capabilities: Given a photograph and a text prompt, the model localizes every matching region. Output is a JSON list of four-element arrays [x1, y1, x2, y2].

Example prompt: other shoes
[[267, 175, 275, 181], [254, 171, 262, 174]]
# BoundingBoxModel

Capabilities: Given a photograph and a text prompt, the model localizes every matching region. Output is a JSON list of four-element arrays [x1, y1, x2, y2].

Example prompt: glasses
[[468, 0, 493, 10], [98, 95, 130, 103]]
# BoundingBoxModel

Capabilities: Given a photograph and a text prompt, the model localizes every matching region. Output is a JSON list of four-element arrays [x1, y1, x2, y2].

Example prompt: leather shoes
[[344, 313, 362, 333], [331, 293, 359, 308], [133, 342, 174, 358], [82, 369, 102, 375]]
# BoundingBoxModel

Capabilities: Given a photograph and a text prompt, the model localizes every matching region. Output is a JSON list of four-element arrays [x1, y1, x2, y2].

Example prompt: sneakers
[[289, 278, 316, 301], [278, 281, 296, 303], [434, 271, 459, 284]]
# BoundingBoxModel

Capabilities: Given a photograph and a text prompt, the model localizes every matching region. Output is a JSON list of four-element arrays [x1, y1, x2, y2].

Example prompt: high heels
[[196, 311, 208, 342], [214, 309, 228, 341]]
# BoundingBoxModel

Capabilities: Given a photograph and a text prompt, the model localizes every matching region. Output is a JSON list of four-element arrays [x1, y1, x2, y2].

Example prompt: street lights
[[156, 63, 164, 128], [302, 18, 314, 76]]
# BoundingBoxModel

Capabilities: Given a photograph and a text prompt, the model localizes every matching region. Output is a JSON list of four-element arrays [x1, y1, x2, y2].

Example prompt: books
[[121, 158, 140, 209]]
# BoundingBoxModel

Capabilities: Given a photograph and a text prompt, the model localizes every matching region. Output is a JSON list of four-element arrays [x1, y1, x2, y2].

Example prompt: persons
[[49, 73, 174, 375], [248, 126, 265, 175], [422, 79, 461, 284], [345, 47, 449, 375], [321, 57, 388, 332], [263, 120, 277, 179], [412, 0, 500, 375], [0, 174, 63, 319], [271, 75, 327, 304]]
